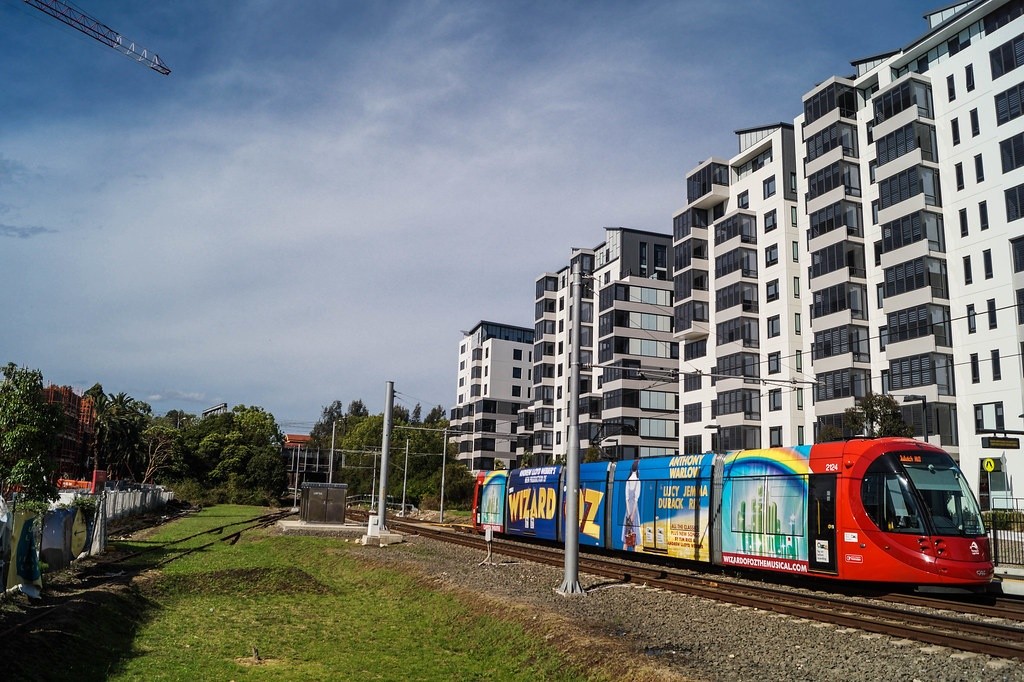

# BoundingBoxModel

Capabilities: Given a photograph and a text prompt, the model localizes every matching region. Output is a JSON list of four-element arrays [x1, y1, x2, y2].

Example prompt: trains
[[473, 437, 994, 586]]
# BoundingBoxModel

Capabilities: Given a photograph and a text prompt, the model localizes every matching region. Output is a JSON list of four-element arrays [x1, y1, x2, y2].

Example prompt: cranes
[[24, 1, 173, 75]]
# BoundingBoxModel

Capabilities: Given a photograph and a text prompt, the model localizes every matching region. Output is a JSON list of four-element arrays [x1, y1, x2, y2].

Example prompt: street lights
[[903, 395, 928, 443], [178, 413, 187, 428], [604, 439, 619, 460], [705, 425, 722, 453]]
